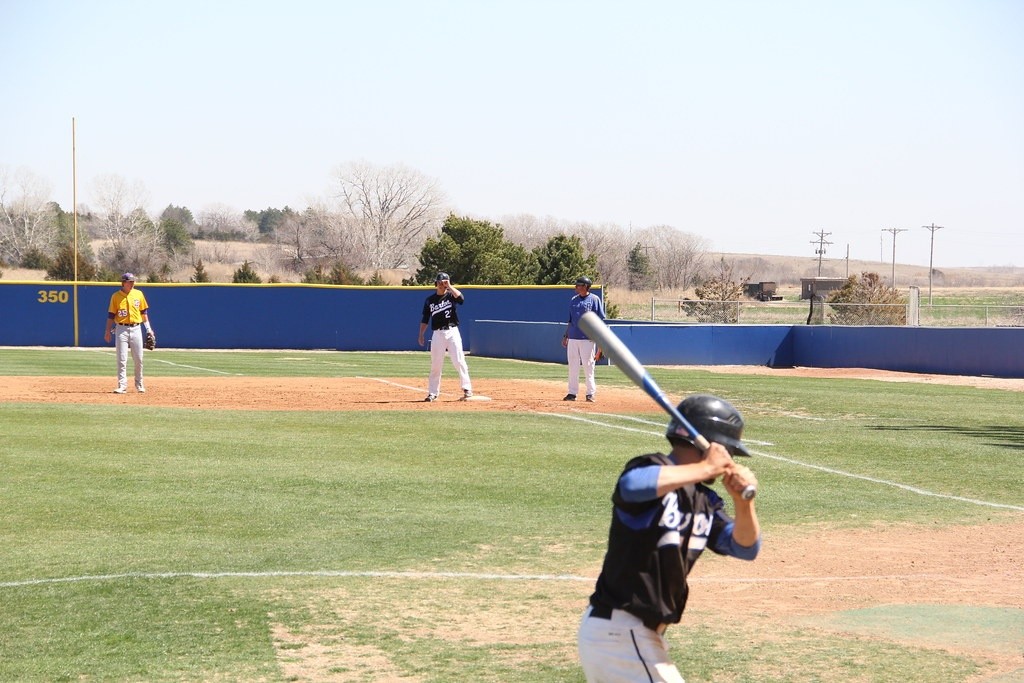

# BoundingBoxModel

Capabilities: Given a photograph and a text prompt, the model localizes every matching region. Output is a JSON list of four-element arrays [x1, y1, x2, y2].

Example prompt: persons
[[104, 273, 155, 393], [577, 392, 763, 683], [562, 276, 605, 403], [418, 272, 473, 401]]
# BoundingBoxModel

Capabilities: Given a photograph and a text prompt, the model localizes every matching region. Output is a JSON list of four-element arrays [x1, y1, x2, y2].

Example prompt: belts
[[440, 325, 457, 329], [589, 607, 667, 636], [119, 323, 139, 327]]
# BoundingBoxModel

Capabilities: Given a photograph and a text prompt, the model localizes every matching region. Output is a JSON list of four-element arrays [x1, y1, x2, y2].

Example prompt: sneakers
[[425, 395, 437, 402], [465, 391, 473, 398], [114, 387, 126, 394], [135, 383, 145, 392], [564, 394, 577, 401], [586, 394, 595, 403]]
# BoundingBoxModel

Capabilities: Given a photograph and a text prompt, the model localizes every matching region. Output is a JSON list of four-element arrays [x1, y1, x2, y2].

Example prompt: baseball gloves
[[145, 328, 157, 350]]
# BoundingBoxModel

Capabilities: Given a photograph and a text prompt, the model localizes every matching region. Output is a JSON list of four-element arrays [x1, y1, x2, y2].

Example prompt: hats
[[122, 273, 138, 281]]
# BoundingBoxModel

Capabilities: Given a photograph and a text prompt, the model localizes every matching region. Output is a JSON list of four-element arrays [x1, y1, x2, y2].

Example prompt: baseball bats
[[576, 309, 756, 501]]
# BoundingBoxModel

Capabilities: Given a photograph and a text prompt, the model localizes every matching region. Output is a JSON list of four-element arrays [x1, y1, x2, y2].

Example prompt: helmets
[[435, 273, 450, 287], [666, 396, 752, 457], [575, 277, 592, 286]]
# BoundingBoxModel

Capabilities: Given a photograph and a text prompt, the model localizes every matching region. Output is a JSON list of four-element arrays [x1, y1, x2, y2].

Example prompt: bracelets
[[564, 334, 568, 338], [419, 336, 424, 337]]
